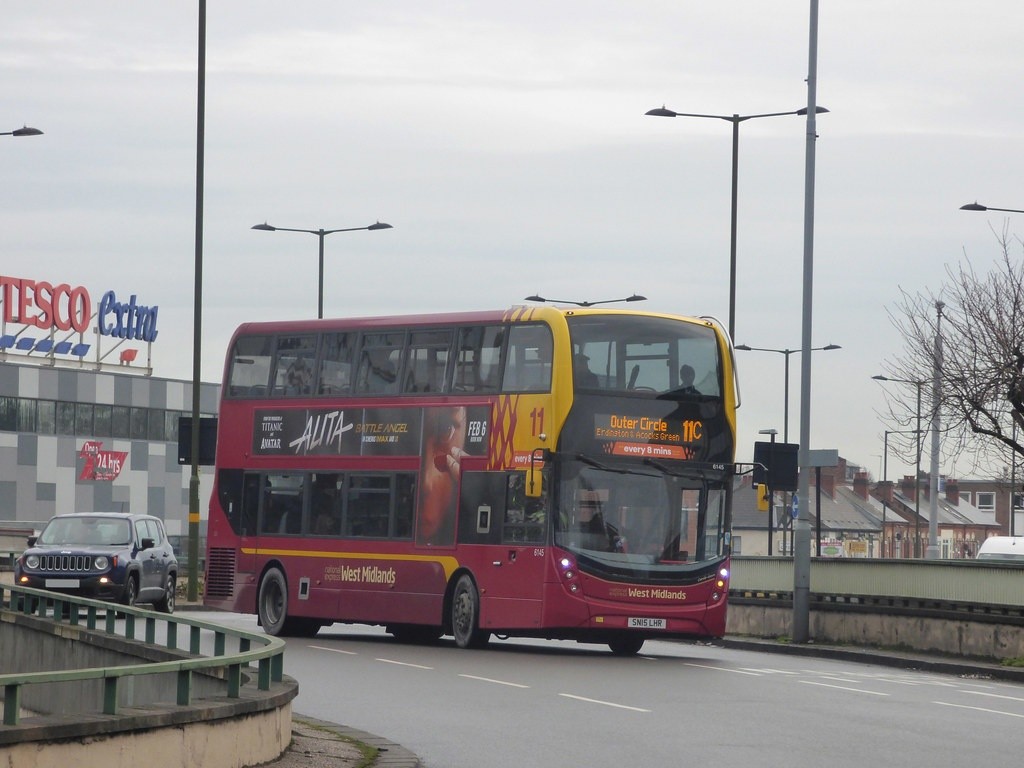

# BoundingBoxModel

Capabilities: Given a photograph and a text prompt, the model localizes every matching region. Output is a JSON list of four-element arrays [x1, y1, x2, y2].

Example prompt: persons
[[534, 497, 568, 529], [677, 365, 701, 394], [422, 407, 470, 538], [576, 353, 598, 386]]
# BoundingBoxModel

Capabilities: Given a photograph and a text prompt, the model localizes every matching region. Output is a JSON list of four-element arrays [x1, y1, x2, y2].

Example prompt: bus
[[200, 304, 770, 656]]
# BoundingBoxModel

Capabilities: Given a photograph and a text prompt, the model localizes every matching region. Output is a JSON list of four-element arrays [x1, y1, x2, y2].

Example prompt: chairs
[[246, 380, 497, 392]]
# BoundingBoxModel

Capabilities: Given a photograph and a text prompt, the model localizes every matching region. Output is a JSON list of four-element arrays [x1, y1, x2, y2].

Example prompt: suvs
[[13, 510, 179, 617]]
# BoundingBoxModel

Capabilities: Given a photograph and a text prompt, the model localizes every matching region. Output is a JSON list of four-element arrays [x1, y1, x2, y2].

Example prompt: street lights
[[880, 429, 926, 560], [758, 429, 778, 556], [643, 105, 830, 566], [734, 344, 843, 559], [872, 375, 963, 559], [250, 222, 394, 320]]
